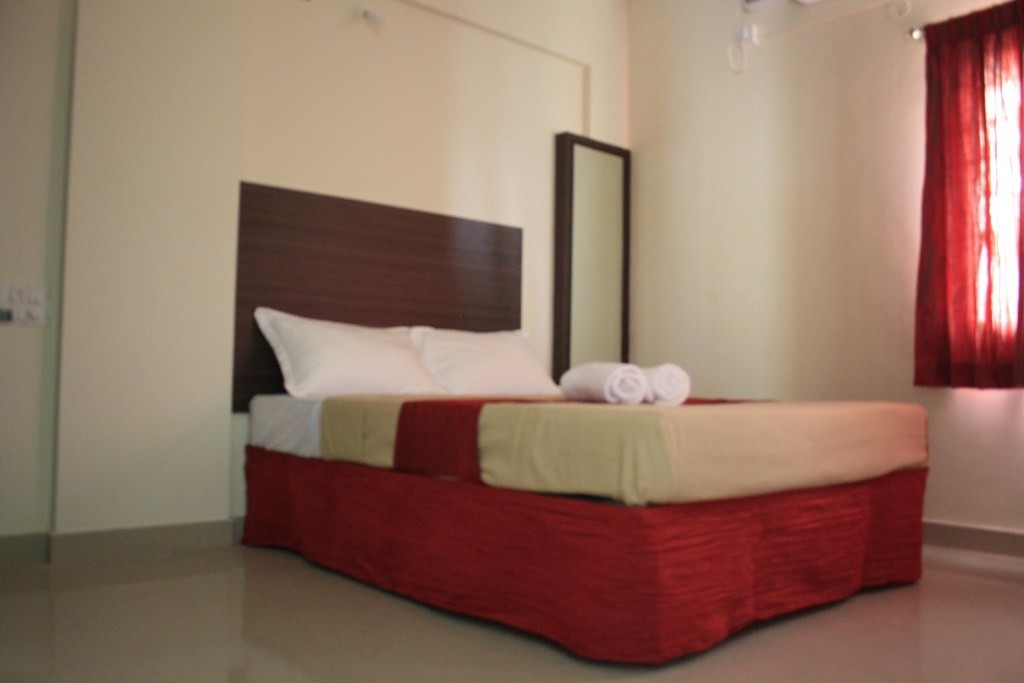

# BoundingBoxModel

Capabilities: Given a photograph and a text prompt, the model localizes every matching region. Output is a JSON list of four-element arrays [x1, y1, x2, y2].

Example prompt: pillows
[[409, 326, 561, 399], [255, 306, 446, 400]]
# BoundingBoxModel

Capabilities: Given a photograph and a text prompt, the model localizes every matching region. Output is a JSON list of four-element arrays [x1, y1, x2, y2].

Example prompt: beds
[[233, 181, 928, 660]]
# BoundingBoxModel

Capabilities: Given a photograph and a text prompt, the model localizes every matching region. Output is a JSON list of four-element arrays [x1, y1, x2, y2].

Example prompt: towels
[[557, 361, 691, 407]]
